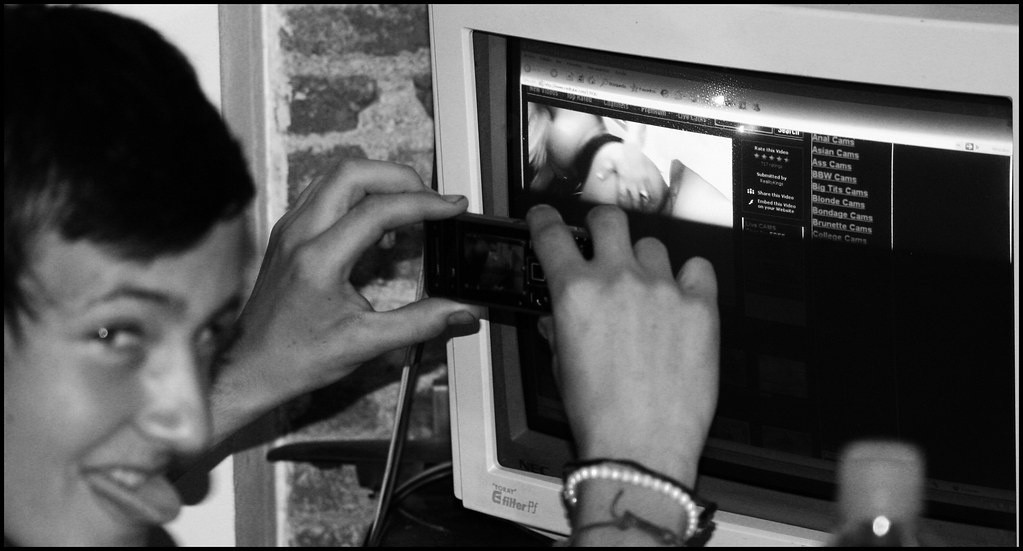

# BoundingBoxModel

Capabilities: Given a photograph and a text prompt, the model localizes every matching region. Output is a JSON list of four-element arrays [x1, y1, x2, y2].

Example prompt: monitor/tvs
[[427, 4, 1019, 547]]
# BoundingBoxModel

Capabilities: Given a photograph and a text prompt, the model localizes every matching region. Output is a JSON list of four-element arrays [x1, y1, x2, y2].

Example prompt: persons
[[527, 100, 670, 211], [3, 0, 723, 550]]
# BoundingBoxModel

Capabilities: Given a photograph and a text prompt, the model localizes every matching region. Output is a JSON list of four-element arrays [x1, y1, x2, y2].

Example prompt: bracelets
[[561, 457, 719, 548]]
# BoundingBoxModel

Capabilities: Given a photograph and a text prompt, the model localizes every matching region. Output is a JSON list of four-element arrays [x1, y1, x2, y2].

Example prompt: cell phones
[[425, 211, 590, 317]]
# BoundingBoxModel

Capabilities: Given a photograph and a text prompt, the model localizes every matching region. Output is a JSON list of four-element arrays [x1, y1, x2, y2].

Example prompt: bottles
[[825, 442, 949, 546]]
